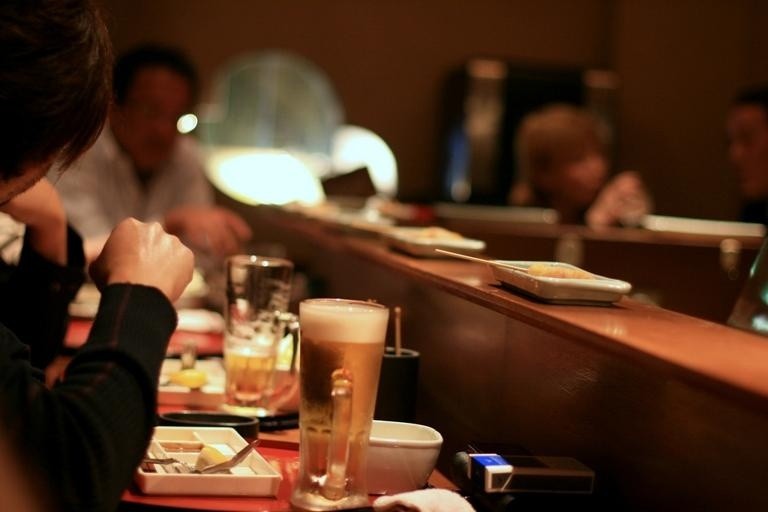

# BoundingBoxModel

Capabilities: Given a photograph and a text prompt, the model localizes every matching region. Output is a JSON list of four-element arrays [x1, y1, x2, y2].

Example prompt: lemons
[[196, 446, 228, 470], [170, 369, 207, 388]]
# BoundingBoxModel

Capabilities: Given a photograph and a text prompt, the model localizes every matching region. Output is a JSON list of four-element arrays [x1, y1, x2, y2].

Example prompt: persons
[[723, 87, 768, 224], [0, 0, 195, 512], [45, 39, 253, 264], [507, 106, 656, 227]]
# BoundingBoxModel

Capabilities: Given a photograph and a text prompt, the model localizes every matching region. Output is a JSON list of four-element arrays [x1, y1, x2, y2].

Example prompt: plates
[[138, 426, 281, 497], [383, 224, 483, 259], [641, 210, 766, 246], [494, 257, 632, 305], [155, 354, 227, 409]]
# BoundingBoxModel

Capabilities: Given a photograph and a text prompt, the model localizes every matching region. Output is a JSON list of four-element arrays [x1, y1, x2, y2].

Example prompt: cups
[[224, 255, 297, 420], [290, 296, 391, 510]]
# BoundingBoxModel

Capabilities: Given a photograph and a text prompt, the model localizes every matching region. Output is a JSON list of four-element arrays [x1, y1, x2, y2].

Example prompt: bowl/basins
[[367, 418, 444, 494], [159, 412, 261, 442]]
